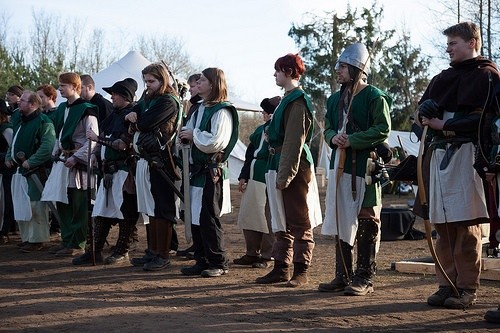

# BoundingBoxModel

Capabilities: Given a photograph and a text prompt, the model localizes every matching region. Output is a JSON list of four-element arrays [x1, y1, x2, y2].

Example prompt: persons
[[413, 22, 500, 309], [0, 54, 311, 287], [318, 42, 392, 295]]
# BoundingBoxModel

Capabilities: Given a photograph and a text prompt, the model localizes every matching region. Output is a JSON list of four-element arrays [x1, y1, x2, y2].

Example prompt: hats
[[102, 78, 138, 102], [8, 84, 24, 97], [338, 43, 370, 76], [260, 96, 281, 114]]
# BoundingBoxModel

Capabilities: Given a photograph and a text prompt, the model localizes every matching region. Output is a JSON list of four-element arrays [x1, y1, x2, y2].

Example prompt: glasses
[[7, 92, 14, 96]]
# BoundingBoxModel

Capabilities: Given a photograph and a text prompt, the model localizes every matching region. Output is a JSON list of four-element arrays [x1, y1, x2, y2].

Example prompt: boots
[[287, 262, 308, 287], [256, 260, 289, 284], [318, 233, 356, 292], [343, 229, 381, 296]]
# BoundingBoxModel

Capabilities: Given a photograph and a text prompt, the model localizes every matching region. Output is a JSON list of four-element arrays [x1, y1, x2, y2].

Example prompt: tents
[[54, 51, 152, 107]]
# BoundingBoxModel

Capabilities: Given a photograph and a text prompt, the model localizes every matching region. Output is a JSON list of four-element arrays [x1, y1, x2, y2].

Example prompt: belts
[[268, 145, 282, 156]]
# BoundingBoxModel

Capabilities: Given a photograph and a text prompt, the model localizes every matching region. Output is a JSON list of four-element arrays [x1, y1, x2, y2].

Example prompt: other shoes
[[142, 256, 170, 271], [132, 249, 159, 265], [484, 307, 500, 323], [252, 259, 275, 268], [181, 262, 209, 275], [186, 251, 195, 260], [176, 245, 197, 256], [16, 238, 129, 265], [443, 288, 477, 309], [427, 287, 453, 306], [201, 263, 229, 277], [233, 255, 259, 264]]
